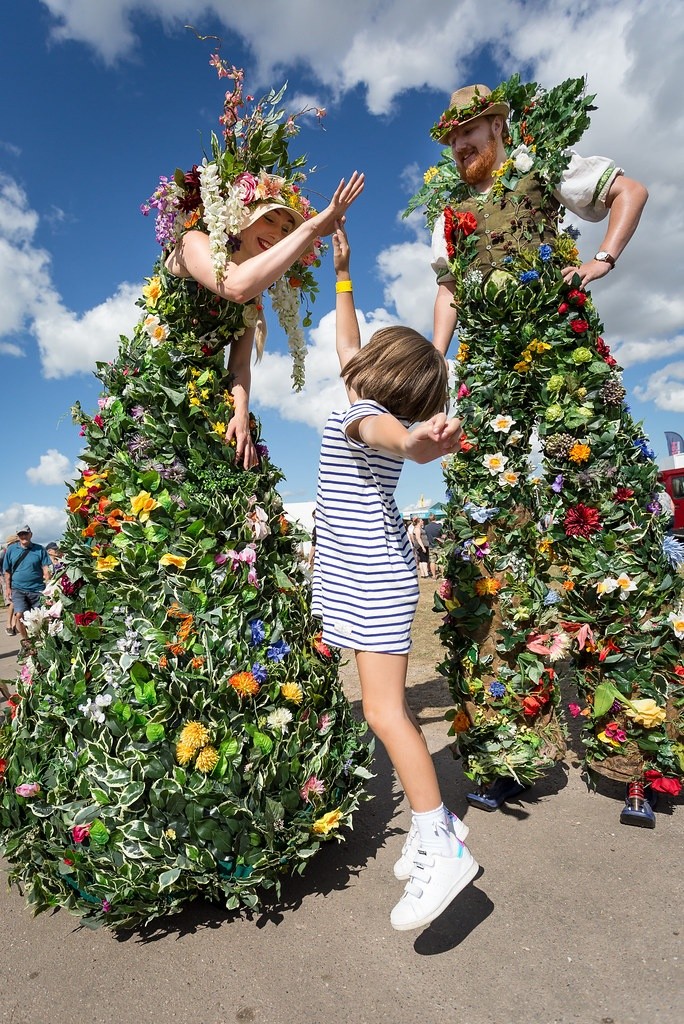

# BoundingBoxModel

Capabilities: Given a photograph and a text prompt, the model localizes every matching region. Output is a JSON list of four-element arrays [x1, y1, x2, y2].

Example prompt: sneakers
[[393, 806, 469, 881], [390, 832, 479, 931]]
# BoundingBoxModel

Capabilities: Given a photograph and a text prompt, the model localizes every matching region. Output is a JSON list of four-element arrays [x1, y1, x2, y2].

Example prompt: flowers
[[396, 72, 684, 798], [0, 20, 380, 936]]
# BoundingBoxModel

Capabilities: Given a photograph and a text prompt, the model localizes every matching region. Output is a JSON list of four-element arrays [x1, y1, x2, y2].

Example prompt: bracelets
[[335, 280, 354, 294]]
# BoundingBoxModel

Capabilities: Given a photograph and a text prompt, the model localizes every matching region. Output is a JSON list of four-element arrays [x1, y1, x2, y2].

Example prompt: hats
[[6, 534, 21, 546], [46, 542, 58, 550], [225, 172, 314, 255], [15, 524, 31, 535], [436, 84, 510, 144]]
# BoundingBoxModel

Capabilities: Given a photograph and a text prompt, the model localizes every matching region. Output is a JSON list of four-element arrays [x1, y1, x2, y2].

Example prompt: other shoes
[[466, 775, 531, 812], [619, 781, 658, 828], [4, 627, 14, 636], [12, 625, 21, 634]]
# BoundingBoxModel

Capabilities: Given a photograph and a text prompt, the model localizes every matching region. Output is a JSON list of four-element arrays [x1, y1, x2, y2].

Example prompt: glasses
[[18, 532, 29, 536]]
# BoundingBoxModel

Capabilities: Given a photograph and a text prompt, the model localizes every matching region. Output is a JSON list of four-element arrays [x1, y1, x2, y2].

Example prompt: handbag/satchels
[[0, 549, 11, 573]]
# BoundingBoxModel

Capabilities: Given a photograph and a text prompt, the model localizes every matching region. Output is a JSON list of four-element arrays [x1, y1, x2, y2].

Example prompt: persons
[[432, 84, 684, 828], [311, 219, 479, 932], [0, 25, 368, 931]]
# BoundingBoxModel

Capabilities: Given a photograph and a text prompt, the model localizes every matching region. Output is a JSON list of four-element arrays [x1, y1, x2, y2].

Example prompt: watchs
[[594, 251, 616, 270]]
